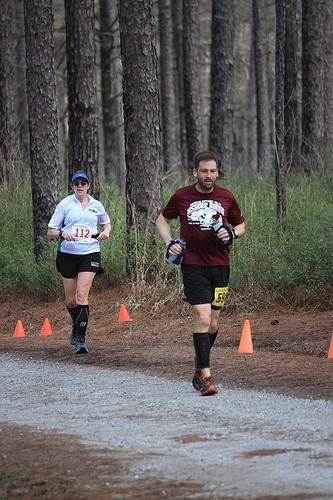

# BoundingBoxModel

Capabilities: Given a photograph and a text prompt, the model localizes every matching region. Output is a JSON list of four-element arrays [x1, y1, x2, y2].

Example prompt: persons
[[156, 152, 245, 395], [46, 171, 112, 353]]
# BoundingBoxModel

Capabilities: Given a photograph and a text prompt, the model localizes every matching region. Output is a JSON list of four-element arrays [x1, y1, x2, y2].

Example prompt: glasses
[[73, 181, 86, 186]]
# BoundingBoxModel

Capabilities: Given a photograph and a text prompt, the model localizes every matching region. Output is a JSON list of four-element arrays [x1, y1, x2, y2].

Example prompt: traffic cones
[[38, 318, 52, 336], [12, 320, 27, 338], [116, 304, 133, 323], [238, 320, 254, 354], [327, 334, 333, 359]]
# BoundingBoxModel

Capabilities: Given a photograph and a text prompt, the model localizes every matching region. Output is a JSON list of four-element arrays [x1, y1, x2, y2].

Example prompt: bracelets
[[59, 231, 64, 238]]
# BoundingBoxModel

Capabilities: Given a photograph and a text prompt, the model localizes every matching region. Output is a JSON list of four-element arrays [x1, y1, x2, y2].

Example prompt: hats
[[72, 172, 89, 181]]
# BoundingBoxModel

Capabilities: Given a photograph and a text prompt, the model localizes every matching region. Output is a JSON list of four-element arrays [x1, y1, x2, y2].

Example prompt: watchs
[[232, 229, 238, 239]]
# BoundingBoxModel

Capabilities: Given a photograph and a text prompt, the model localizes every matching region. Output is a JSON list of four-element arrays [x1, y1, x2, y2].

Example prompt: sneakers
[[201, 376, 218, 395], [192, 356, 202, 390], [76, 341, 89, 354], [70, 324, 77, 345]]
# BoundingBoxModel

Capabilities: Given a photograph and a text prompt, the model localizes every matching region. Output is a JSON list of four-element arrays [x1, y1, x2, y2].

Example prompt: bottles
[[167, 239, 186, 265]]
